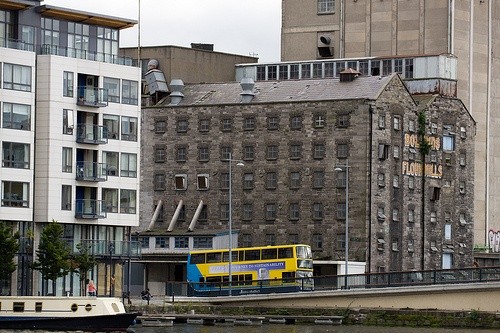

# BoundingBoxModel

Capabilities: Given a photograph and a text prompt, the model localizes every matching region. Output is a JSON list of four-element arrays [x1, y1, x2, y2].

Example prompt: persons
[[88, 279, 97, 296], [142, 288, 153, 300]]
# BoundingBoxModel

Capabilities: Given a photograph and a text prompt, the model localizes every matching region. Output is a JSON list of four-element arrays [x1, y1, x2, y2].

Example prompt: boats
[[0, 291, 143, 332]]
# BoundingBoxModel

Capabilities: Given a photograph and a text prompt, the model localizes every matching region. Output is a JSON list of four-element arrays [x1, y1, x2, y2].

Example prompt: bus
[[185, 243, 315, 297]]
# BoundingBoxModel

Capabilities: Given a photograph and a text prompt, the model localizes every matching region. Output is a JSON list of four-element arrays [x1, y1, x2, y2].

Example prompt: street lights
[[221, 152, 245, 297], [334, 159, 349, 289]]
